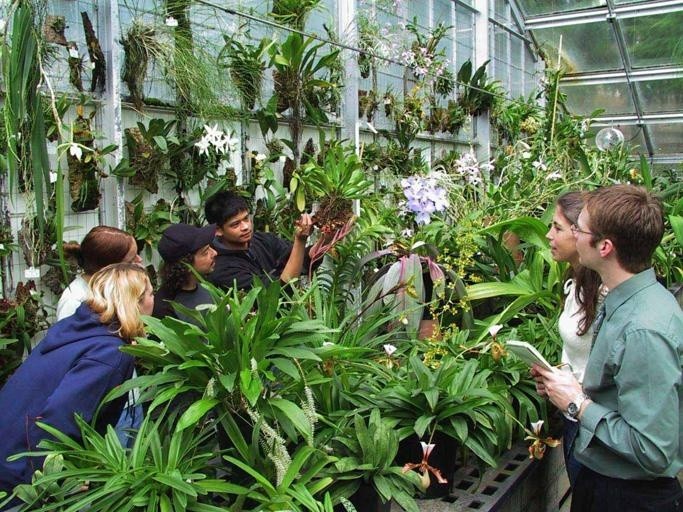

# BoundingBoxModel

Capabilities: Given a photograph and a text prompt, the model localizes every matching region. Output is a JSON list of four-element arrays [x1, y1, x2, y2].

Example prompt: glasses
[[570, 224, 593, 239]]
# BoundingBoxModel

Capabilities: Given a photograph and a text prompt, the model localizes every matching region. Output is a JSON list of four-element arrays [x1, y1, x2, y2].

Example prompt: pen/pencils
[[527, 363, 564, 379]]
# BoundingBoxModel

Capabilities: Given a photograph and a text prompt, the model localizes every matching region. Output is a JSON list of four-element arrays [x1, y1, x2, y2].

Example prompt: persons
[[56, 225, 144, 451], [204, 191, 331, 319], [0, 262, 155, 507], [152, 223, 218, 346], [528, 184, 683, 512]]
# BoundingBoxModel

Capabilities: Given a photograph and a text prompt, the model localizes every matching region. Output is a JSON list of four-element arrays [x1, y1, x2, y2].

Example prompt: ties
[[589, 302, 606, 356]]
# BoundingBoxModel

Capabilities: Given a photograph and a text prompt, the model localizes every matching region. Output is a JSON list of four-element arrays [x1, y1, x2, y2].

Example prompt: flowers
[[396, 169, 455, 231], [351, 0, 453, 81]]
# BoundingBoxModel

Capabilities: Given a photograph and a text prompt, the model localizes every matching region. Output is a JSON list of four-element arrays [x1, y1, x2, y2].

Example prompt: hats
[[156, 222, 218, 264]]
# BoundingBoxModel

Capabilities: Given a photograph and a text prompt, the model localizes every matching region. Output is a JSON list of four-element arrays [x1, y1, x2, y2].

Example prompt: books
[[506, 339, 557, 376]]
